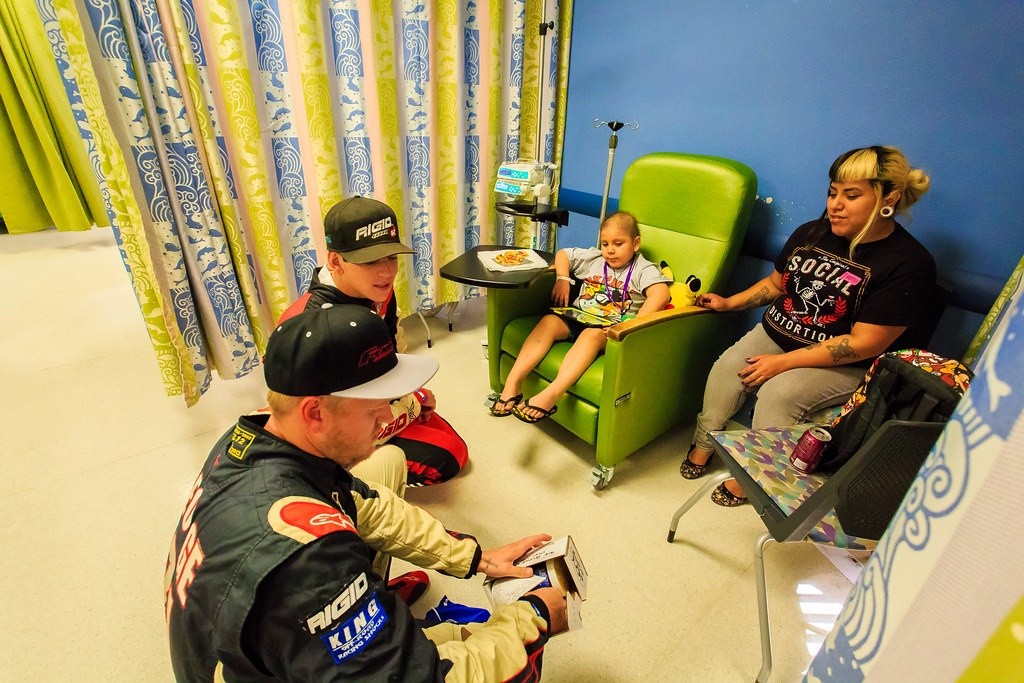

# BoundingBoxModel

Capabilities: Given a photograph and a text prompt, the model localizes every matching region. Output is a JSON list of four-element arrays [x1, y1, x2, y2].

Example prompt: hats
[[323, 194, 418, 265], [262, 303, 440, 400]]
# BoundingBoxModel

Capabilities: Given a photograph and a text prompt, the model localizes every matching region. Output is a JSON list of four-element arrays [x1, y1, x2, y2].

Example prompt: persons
[[276, 196, 468, 488], [679, 143, 937, 506], [492, 212, 674, 424], [164, 306, 569, 682]]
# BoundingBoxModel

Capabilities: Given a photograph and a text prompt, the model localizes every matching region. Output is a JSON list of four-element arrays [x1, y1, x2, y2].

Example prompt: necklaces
[[612, 264, 631, 303]]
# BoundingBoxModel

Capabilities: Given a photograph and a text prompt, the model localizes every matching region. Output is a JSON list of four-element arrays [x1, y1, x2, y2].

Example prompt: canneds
[[789, 427, 832, 474]]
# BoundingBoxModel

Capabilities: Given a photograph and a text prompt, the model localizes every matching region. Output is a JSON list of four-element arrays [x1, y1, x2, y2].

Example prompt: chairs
[[485, 153, 759, 492], [417, 301, 460, 348], [732, 392, 847, 429], [667, 419, 948, 683]]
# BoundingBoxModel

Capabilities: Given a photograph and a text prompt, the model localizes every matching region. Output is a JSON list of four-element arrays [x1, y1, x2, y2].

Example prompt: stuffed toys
[[660, 260, 701, 311]]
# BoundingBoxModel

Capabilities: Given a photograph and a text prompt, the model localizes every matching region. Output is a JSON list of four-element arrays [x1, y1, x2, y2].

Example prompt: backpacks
[[823, 347, 976, 541]]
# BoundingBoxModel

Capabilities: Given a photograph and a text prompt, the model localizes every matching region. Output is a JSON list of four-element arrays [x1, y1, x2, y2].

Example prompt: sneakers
[[680, 444, 716, 479], [710, 480, 749, 507]]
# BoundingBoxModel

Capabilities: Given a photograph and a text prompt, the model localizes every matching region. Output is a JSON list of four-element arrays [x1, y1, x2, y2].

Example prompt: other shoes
[[388, 570, 430, 607]]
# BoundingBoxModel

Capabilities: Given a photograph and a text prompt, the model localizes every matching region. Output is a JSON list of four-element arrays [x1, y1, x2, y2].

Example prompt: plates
[[492, 257, 534, 267]]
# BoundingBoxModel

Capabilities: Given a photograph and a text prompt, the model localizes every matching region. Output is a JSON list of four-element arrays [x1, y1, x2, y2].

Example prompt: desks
[[437, 244, 556, 288]]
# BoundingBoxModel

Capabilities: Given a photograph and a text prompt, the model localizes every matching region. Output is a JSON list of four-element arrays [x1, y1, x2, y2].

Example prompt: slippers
[[511, 398, 558, 424], [492, 393, 523, 418]]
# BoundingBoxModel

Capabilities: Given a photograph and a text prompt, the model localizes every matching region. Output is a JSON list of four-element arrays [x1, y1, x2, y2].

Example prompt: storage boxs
[[482, 534, 588, 639]]
